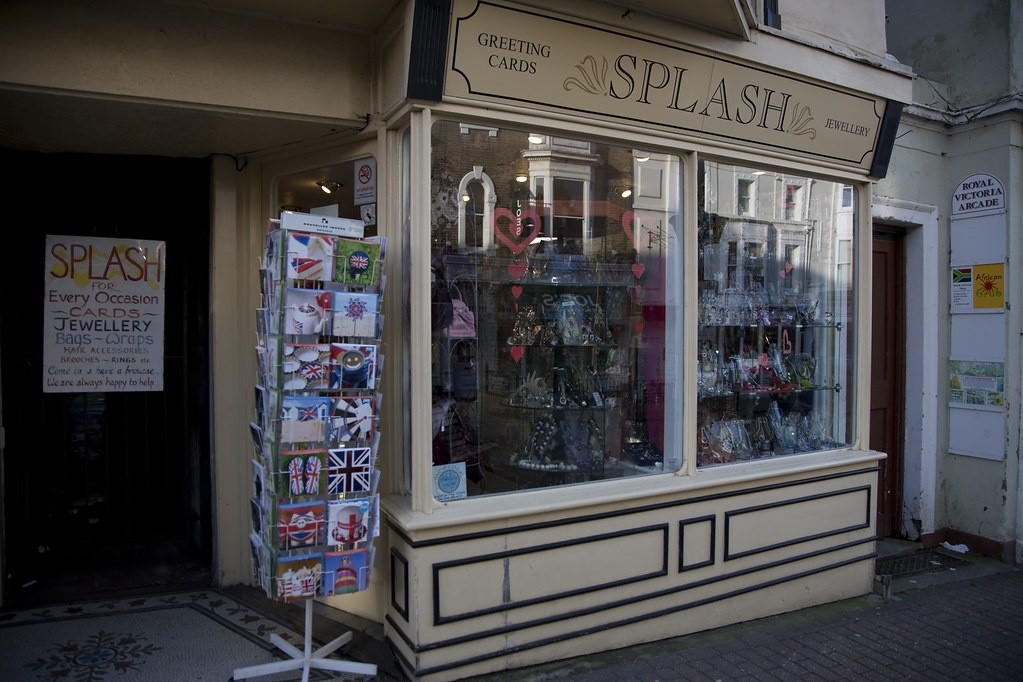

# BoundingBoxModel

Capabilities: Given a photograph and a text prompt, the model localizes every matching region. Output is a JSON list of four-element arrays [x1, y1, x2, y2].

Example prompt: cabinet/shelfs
[[698, 286, 841, 463], [230, 211, 387, 682], [500, 241, 619, 489], [432, 159, 486, 480]]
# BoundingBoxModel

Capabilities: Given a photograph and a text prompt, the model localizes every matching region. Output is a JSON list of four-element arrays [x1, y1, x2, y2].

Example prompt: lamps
[[316, 181, 342, 194]]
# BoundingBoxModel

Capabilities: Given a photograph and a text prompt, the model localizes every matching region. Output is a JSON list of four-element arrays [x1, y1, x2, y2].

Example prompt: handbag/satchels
[[441, 282, 477, 340], [431, 269, 453, 331], [453, 359, 477, 402], [432, 397, 451, 439]]
